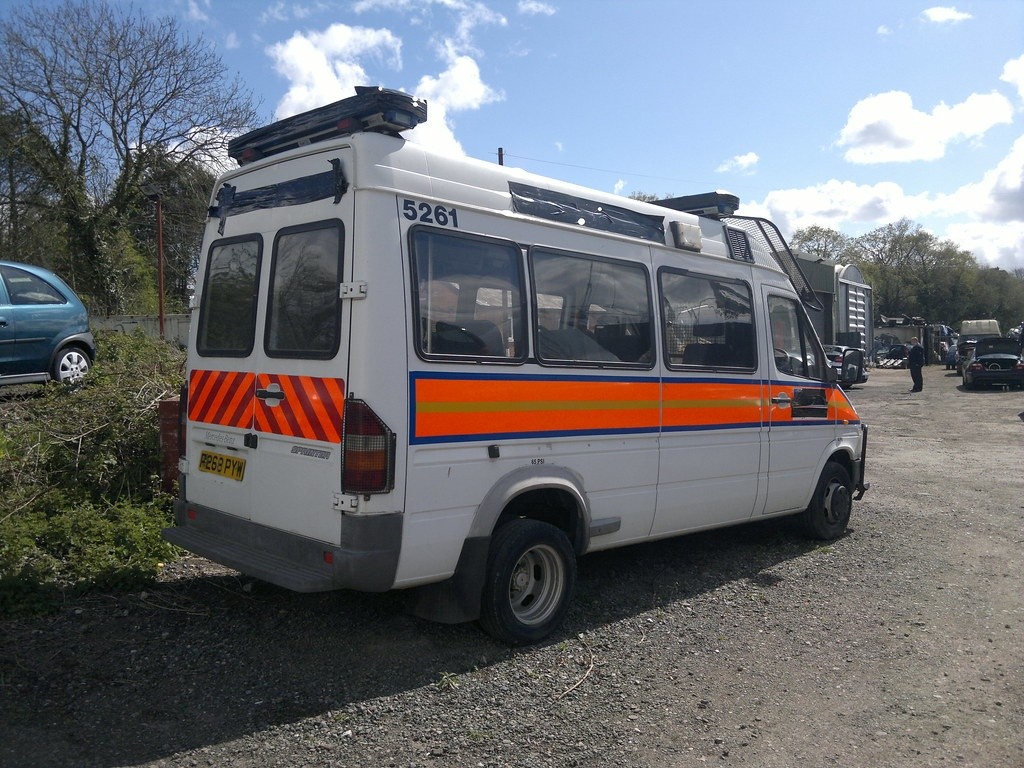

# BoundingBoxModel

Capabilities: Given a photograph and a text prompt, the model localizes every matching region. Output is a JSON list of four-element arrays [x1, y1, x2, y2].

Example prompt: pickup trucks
[[876, 343, 926, 369]]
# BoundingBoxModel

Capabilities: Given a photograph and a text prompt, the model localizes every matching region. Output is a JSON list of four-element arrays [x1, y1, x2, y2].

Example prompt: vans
[[959, 320, 1003, 341], [158, 76, 871, 646]]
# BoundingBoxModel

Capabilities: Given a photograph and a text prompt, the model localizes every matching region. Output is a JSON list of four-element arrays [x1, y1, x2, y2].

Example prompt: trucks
[[873, 324, 959, 354]]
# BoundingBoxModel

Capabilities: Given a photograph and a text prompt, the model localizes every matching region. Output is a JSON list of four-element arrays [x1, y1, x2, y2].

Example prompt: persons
[[907, 336, 927, 393]]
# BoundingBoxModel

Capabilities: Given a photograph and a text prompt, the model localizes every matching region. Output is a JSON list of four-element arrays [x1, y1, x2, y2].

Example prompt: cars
[[822, 344, 868, 384], [956, 341, 977, 374], [945, 345, 957, 370], [0, 260, 96, 387], [961, 337, 1024, 390]]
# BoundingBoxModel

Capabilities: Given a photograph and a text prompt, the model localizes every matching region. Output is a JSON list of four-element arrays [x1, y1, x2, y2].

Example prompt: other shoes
[[909, 389, 922, 392]]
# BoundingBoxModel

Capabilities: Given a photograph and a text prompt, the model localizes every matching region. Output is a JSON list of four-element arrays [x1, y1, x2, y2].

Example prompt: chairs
[[436, 320, 505, 358]]
